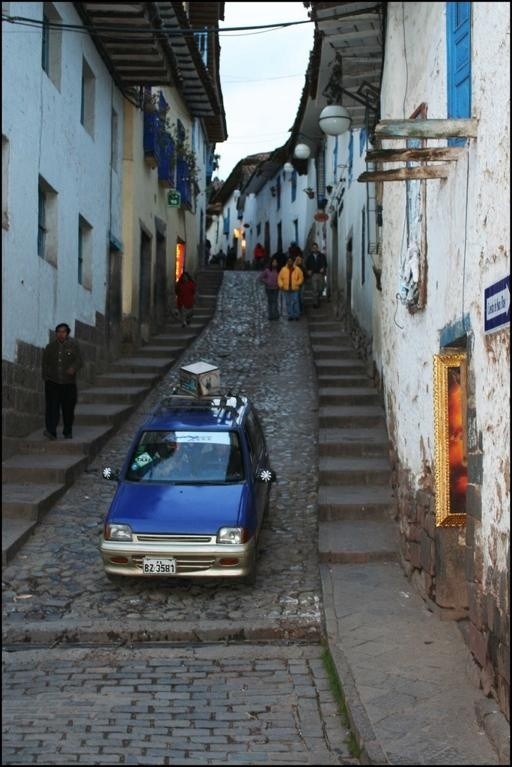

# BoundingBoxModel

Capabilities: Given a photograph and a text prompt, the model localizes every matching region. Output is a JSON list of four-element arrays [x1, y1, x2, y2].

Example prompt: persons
[[42, 323, 84, 439], [174, 272, 197, 327], [206, 240, 235, 270], [254, 241, 328, 322]]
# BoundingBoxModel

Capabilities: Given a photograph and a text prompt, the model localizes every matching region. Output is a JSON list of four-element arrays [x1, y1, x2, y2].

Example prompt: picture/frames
[[433, 352, 468, 527]]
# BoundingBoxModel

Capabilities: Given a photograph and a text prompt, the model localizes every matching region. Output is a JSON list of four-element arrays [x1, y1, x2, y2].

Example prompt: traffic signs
[[482, 274, 511, 335]]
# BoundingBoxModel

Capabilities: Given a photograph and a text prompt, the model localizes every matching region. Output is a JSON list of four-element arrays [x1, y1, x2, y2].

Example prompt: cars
[[95, 390, 278, 587]]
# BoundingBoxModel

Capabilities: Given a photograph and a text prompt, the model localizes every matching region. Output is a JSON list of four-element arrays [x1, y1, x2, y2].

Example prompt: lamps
[[283, 161, 294, 172], [294, 133, 319, 159], [318, 81, 380, 136]]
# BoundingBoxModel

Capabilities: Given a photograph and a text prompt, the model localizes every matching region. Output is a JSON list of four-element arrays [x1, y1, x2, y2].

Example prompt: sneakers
[[181, 318, 191, 328], [43, 429, 57, 441], [62, 428, 72, 439], [268, 303, 319, 322]]
